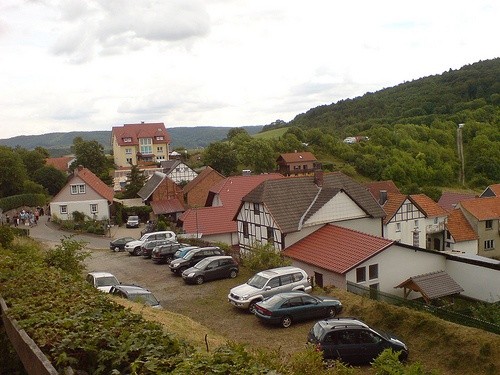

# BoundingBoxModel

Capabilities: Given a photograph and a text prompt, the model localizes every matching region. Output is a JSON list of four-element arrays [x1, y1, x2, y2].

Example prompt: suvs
[[170, 247, 226, 276], [227, 265, 313, 315], [124, 231, 179, 256], [306, 316, 409, 370]]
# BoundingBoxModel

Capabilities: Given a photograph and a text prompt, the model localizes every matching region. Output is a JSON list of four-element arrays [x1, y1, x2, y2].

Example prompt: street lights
[[457, 123, 467, 189]]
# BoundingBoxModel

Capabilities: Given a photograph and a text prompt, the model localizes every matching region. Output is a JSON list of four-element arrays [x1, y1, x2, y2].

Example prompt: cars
[[108, 284, 163, 311], [86, 272, 123, 293], [109, 237, 136, 252], [254, 289, 344, 328], [142, 240, 200, 264], [181, 256, 240, 286], [125, 216, 141, 228]]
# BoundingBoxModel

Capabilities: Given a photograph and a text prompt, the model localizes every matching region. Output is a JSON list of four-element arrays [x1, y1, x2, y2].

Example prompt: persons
[[5, 204, 48, 228]]
